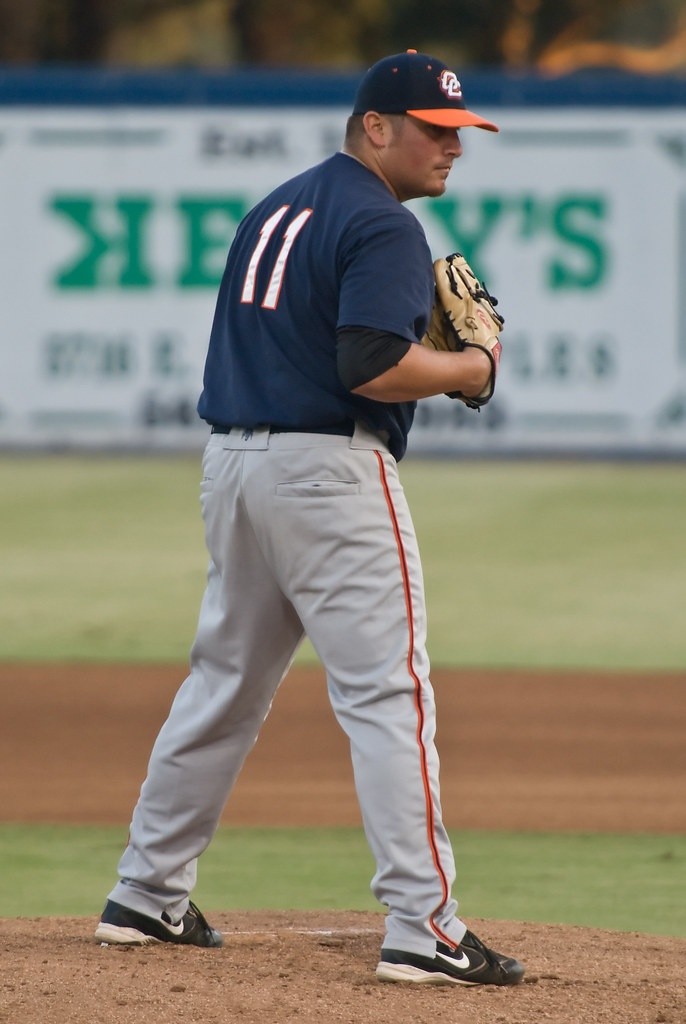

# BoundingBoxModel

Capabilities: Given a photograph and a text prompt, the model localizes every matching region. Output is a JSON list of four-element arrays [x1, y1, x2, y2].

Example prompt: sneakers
[[375, 926, 526, 988], [92, 899, 224, 949]]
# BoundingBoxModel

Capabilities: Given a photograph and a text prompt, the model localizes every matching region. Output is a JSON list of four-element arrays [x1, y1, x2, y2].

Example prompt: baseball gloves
[[420, 253, 505, 412]]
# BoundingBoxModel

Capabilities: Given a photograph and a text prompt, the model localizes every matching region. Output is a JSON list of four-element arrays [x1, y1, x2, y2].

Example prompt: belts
[[209, 415, 355, 437]]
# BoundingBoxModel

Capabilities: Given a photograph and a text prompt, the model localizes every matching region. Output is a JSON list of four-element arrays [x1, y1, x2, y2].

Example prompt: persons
[[92, 0, 686, 80], [96, 50, 525, 988]]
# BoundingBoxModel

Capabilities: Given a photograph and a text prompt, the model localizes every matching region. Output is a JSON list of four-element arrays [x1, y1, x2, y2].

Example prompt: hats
[[352, 48, 499, 134]]
